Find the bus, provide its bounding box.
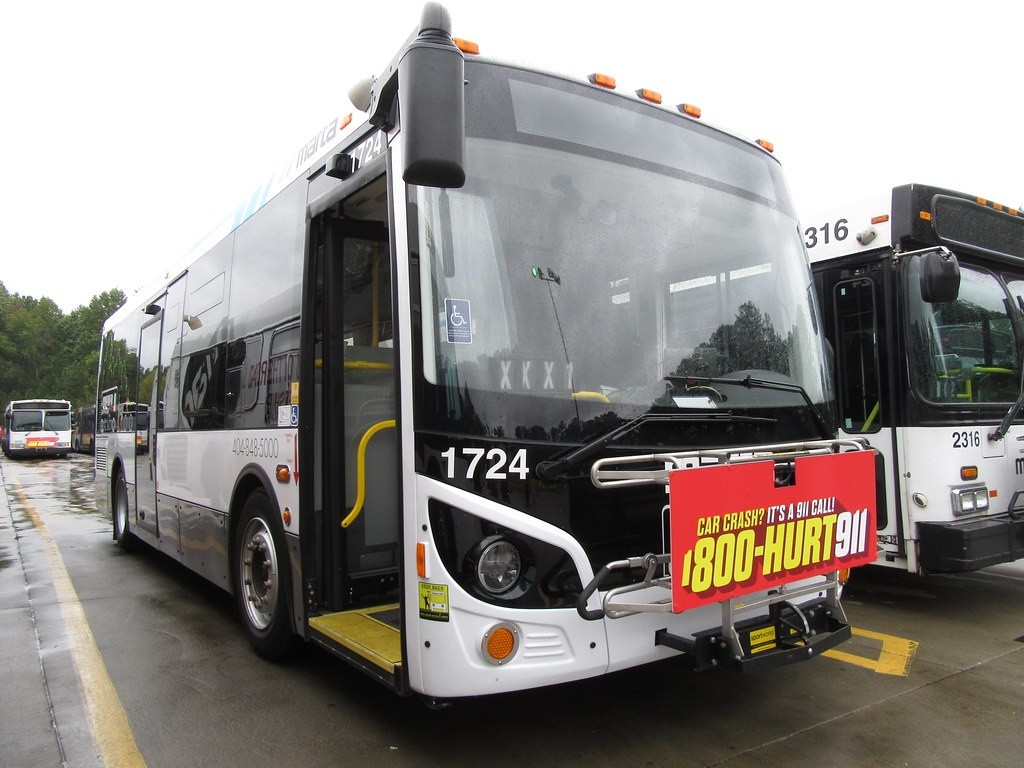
[94,1,877,715]
[3,399,77,459]
[117,403,147,454]
[72,402,96,457]
[611,183,1024,597]
